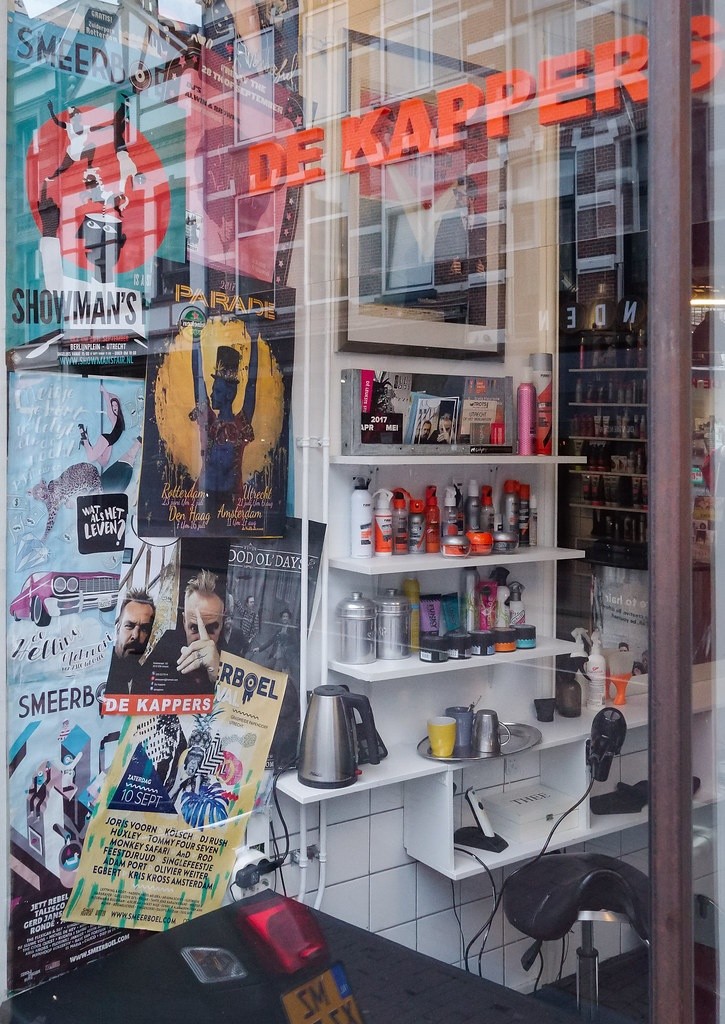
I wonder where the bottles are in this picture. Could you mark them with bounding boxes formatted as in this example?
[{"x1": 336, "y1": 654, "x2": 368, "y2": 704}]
[
  {"x1": 333, "y1": 575, "x2": 421, "y2": 666},
  {"x1": 459, "y1": 567, "x2": 480, "y2": 632},
  {"x1": 517, "y1": 366, "x2": 536, "y2": 456}
]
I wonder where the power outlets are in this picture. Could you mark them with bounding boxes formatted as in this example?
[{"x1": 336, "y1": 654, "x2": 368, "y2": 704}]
[{"x1": 231, "y1": 848, "x2": 274, "y2": 899}]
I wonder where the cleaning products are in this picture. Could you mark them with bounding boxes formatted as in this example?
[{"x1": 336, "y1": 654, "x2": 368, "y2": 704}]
[
  {"x1": 569, "y1": 322, "x2": 649, "y2": 545},
  {"x1": 349, "y1": 464, "x2": 538, "y2": 559},
  {"x1": 514, "y1": 352, "x2": 554, "y2": 456}
]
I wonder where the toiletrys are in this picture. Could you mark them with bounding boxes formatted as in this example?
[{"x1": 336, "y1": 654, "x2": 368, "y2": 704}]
[
  {"x1": 401, "y1": 565, "x2": 537, "y2": 664},
  {"x1": 555, "y1": 626, "x2": 635, "y2": 718}
]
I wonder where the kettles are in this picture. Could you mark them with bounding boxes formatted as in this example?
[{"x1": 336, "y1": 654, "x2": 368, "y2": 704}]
[{"x1": 298, "y1": 685, "x2": 380, "y2": 789}]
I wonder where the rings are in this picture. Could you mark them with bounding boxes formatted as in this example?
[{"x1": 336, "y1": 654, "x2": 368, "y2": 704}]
[
  {"x1": 197, "y1": 649, "x2": 201, "y2": 659},
  {"x1": 198, "y1": 658, "x2": 203, "y2": 667}
]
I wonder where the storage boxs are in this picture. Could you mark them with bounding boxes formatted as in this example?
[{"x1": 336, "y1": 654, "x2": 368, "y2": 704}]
[{"x1": 480, "y1": 783, "x2": 582, "y2": 844}]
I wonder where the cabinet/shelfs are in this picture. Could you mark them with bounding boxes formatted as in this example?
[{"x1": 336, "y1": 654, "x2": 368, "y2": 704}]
[{"x1": 273, "y1": 362, "x2": 715, "y2": 885}]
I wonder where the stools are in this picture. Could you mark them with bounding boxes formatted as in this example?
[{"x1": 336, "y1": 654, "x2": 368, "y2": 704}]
[{"x1": 505, "y1": 845, "x2": 654, "y2": 1019}]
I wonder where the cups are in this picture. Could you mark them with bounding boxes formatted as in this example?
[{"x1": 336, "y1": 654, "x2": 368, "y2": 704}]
[
  {"x1": 471, "y1": 709, "x2": 511, "y2": 753},
  {"x1": 427, "y1": 716, "x2": 456, "y2": 757},
  {"x1": 445, "y1": 706, "x2": 477, "y2": 747}
]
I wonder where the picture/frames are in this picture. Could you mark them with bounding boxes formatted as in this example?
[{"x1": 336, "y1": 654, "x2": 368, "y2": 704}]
[{"x1": 334, "y1": 25, "x2": 510, "y2": 366}]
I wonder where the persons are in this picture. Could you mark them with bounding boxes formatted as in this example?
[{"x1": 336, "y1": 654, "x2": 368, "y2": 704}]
[
  {"x1": 414, "y1": 417, "x2": 454, "y2": 444},
  {"x1": 101, "y1": 569, "x2": 298, "y2": 697}
]
